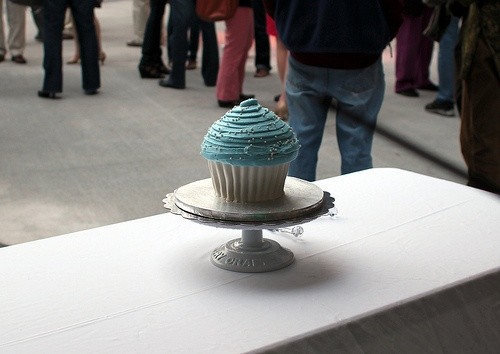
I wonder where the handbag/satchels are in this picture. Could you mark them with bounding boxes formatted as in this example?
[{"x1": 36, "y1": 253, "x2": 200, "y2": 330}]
[
  {"x1": 195, "y1": 0, "x2": 238, "y2": 22},
  {"x1": 421, "y1": 4, "x2": 453, "y2": 42}
]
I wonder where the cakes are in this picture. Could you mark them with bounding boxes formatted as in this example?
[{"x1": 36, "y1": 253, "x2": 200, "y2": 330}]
[{"x1": 200, "y1": 98, "x2": 301, "y2": 202}]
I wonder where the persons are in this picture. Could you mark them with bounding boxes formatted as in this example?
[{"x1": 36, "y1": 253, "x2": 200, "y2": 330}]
[
  {"x1": 267, "y1": 0, "x2": 405, "y2": 182},
  {"x1": 167, "y1": 0, "x2": 200, "y2": 69},
  {"x1": 460, "y1": 0, "x2": 500, "y2": 194},
  {"x1": 11, "y1": 0, "x2": 103, "y2": 98},
  {"x1": 266, "y1": 12, "x2": 290, "y2": 121},
  {"x1": 65, "y1": 7, "x2": 107, "y2": 65},
  {"x1": 424, "y1": 0, "x2": 460, "y2": 116},
  {"x1": 239, "y1": 0, "x2": 272, "y2": 78},
  {"x1": 36, "y1": 7, "x2": 75, "y2": 40},
  {"x1": 138, "y1": 0, "x2": 172, "y2": 79},
  {"x1": 159, "y1": 0, "x2": 220, "y2": 89},
  {"x1": 216, "y1": 0, "x2": 255, "y2": 108},
  {"x1": 395, "y1": 0, "x2": 440, "y2": 98},
  {"x1": 0, "y1": 0, "x2": 29, "y2": 64},
  {"x1": 126, "y1": 0, "x2": 167, "y2": 47}
]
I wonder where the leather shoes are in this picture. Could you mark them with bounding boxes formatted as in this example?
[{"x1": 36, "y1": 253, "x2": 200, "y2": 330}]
[
  {"x1": 36, "y1": 33, "x2": 74, "y2": 41},
  {"x1": 125, "y1": 38, "x2": 145, "y2": 47},
  {"x1": 218, "y1": 93, "x2": 257, "y2": 107},
  {"x1": 0, "y1": 53, "x2": 27, "y2": 63},
  {"x1": 254, "y1": 67, "x2": 268, "y2": 77},
  {"x1": 274, "y1": 92, "x2": 283, "y2": 100}
]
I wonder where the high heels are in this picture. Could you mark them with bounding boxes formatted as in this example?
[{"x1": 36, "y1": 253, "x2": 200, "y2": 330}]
[
  {"x1": 64, "y1": 55, "x2": 85, "y2": 65},
  {"x1": 272, "y1": 103, "x2": 291, "y2": 121},
  {"x1": 96, "y1": 52, "x2": 106, "y2": 65}
]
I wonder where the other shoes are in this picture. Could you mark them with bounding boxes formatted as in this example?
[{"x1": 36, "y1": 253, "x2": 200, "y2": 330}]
[
  {"x1": 396, "y1": 88, "x2": 418, "y2": 97},
  {"x1": 38, "y1": 87, "x2": 56, "y2": 98},
  {"x1": 418, "y1": 84, "x2": 439, "y2": 92},
  {"x1": 157, "y1": 78, "x2": 186, "y2": 88},
  {"x1": 187, "y1": 61, "x2": 197, "y2": 69},
  {"x1": 140, "y1": 60, "x2": 170, "y2": 78}
]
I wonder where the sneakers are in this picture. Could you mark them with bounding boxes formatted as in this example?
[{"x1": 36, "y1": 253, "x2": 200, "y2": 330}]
[{"x1": 425, "y1": 100, "x2": 454, "y2": 116}]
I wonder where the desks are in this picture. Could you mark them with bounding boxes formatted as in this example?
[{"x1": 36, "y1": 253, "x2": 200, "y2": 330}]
[{"x1": 0, "y1": 167, "x2": 500, "y2": 354}]
